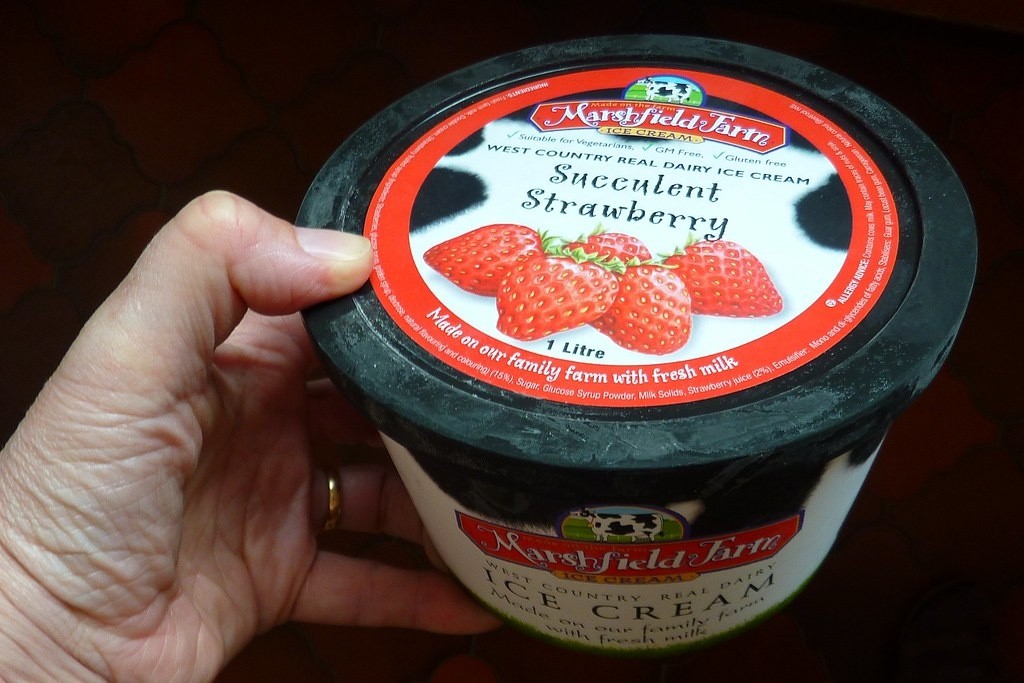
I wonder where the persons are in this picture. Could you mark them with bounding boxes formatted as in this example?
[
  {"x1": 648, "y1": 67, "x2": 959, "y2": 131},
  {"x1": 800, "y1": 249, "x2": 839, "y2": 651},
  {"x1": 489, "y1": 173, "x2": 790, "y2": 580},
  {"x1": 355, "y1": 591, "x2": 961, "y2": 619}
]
[{"x1": 0, "y1": 189, "x2": 503, "y2": 683}]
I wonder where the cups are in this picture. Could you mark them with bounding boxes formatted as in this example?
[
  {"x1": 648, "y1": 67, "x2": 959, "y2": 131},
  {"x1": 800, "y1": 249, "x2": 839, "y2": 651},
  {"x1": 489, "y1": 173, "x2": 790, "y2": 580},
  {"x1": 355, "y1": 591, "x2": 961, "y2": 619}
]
[{"x1": 296, "y1": 33, "x2": 978, "y2": 658}]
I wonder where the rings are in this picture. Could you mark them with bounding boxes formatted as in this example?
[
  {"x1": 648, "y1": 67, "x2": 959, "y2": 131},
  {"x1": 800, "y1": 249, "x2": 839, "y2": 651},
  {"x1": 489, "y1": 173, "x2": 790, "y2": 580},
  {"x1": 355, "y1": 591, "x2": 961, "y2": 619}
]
[{"x1": 326, "y1": 467, "x2": 340, "y2": 531}]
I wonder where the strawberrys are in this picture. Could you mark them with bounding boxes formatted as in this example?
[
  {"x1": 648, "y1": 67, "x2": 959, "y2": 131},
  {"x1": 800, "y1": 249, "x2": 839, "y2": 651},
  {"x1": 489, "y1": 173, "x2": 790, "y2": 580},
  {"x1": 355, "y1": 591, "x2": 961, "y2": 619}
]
[{"x1": 422, "y1": 224, "x2": 784, "y2": 356}]
